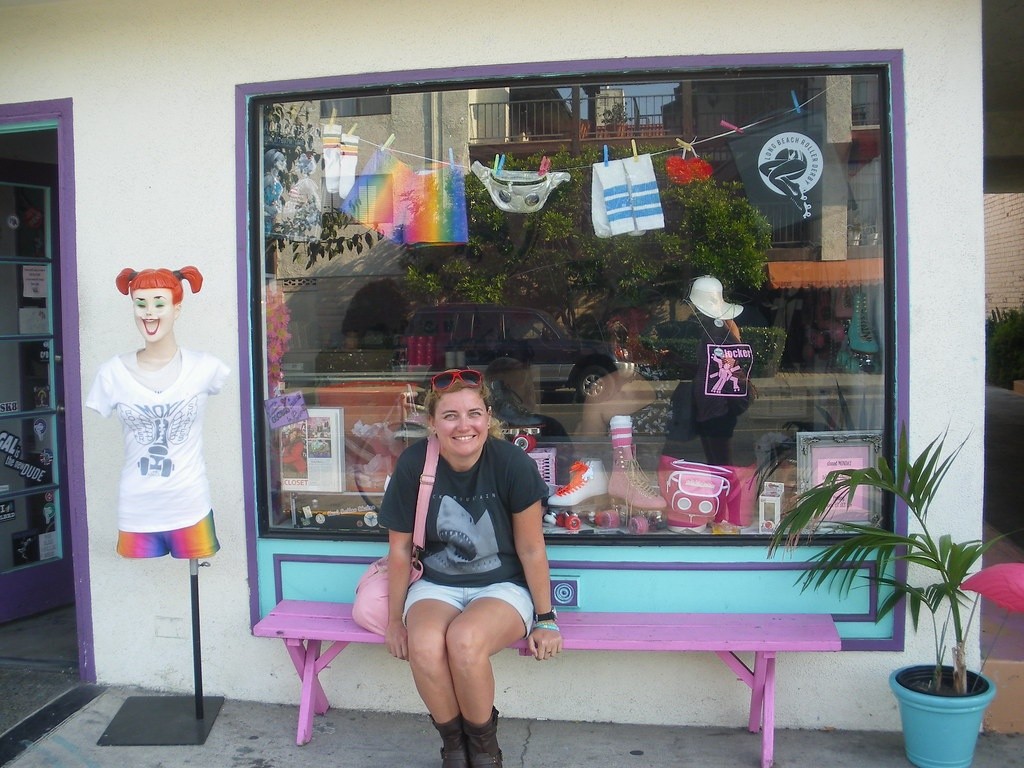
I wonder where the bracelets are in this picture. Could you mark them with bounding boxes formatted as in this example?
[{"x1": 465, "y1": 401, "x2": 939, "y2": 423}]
[{"x1": 533, "y1": 622, "x2": 559, "y2": 631}]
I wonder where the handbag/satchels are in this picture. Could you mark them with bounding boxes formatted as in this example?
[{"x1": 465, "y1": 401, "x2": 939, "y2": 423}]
[
  {"x1": 352, "y1": 555, "x2": 423, "y2": 636},
  {"x1": 657, "y1": 453, "x2": 757, "y2": 527}
]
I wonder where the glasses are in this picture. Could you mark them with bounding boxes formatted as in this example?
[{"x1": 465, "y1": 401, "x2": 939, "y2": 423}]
[{"x1": 431, "y1": 370, "x2": 482, "y2": 392}]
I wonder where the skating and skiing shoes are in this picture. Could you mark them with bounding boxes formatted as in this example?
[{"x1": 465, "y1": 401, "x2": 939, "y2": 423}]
[
  {"x1": 598, "y1": 444, "x2": 667, "y2": 533},
  {"x1": 545, "y1": 459, "x2": 610, "y2": 530}
]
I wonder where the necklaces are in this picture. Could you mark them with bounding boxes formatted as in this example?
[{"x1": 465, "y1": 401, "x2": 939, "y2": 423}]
[{"x1": 683, "y1": 297, "x2": 735, "y2": 356}]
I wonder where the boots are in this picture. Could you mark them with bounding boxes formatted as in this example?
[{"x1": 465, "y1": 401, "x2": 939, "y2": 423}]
[{"x1": 428, "y1": 706, "x2": 504, "y2": 768}]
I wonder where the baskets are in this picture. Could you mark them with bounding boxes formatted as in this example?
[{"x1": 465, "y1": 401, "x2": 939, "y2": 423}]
[{"x1": 526, "y1": 447, "x2": 558, "y2": 485}]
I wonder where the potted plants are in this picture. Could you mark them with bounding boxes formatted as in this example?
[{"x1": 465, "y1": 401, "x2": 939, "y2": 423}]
[{"x1": 762, "y1": 428, "x2": 1024, "y2": 768}]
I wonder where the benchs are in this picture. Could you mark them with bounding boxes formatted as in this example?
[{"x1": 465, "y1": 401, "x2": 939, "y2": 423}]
[{"x1": 252, "y1": 597, "x2": 845, "y2": 768}]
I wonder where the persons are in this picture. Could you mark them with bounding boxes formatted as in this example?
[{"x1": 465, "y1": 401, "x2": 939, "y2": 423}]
[
  {"x1": 484, "y1": 359, "x2": 574, "y2": 485},
  {"x1": 579, "y1": 309, "x2": 692, "y2": 470},
  {"x1": 98, "y1": 266, "x2": 233, "y2": 559},
  {"x1": 263, "y1": 148, "x2": 286, "y2": 234},
  {"x1": 692, "y1": 290, "x2": 744, "y2": 532},
  {"x1": 284, "y1": 153, "x2": 320, "y2": 236},
  {"x1": 342, "y1": 274, "x2": 414, "y2": 347},
  {"x1": 376, "y1": 370, "x2": 563, "y2": 768}
]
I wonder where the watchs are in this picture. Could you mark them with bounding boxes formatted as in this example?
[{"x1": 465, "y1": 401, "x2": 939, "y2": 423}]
[{"x1": 534, "y1": 606, "x2": 557, "y2": 621}]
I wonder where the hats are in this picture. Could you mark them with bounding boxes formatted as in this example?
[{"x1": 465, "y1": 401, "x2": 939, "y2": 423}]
[{"x1": 688, "y1": 276, "x2": 744, "y2": 320}]
